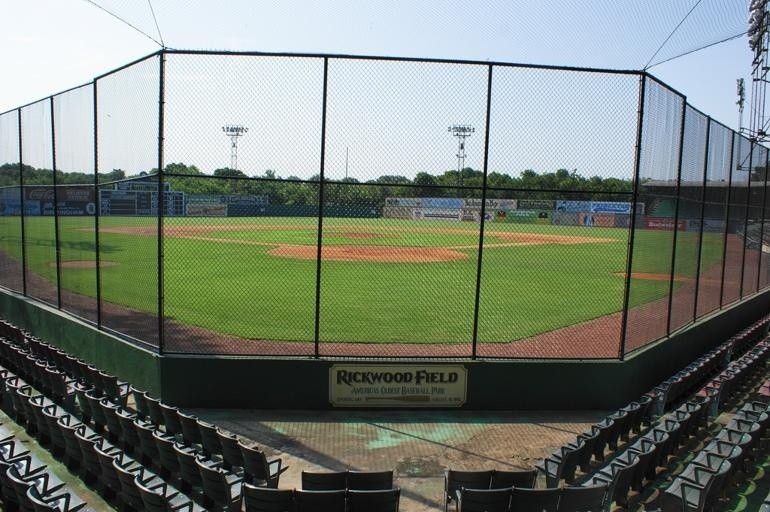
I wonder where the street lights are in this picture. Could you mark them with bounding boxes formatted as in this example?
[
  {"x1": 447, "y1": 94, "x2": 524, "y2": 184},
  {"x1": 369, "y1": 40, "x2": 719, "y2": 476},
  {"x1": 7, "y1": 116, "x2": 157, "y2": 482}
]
[
  {"x1": 222, "y1": 126, "x2": 248, "y2": 172},
  {"x1": 736, "y1": 78, "x2": 745, "y2": 172},
  {"x1": 447, "y1": 124, "x2": 475, "y2": 198}
]
